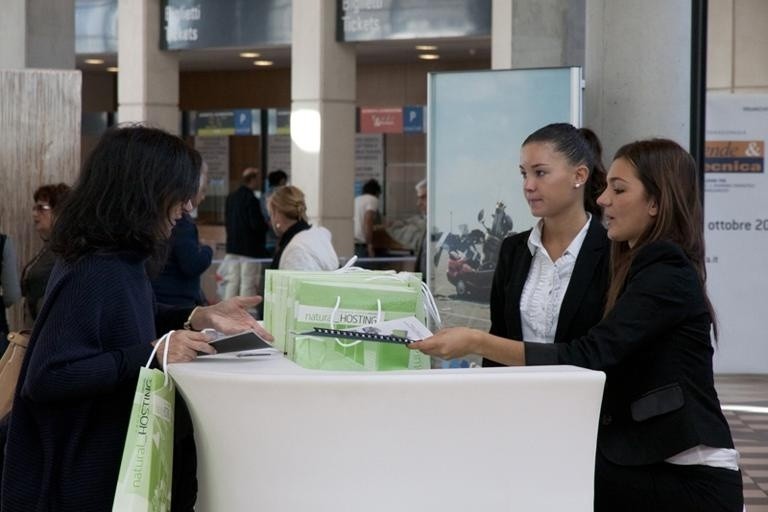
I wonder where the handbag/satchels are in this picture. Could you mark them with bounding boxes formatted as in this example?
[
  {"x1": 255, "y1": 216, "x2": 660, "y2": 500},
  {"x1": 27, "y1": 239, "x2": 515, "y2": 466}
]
[
  {"x1": 111, "y1": 331, "x2": 175, "y2": 511},
  {"x1": 0, "y1": 328, "x2": 34, "y2": 419},
  {"x1": 262, "y1": 255, "x2": 442, "y2": 371}
]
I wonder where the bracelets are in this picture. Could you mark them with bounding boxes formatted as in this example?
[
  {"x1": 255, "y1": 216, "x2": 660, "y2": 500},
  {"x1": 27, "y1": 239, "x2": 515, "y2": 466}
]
[{"x1": 183, "y1": 302, "x2": 202, "y2": 333}]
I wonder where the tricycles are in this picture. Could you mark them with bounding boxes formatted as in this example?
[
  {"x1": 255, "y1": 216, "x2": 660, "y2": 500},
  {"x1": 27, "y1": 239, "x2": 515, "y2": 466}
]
[{"x1": 446, "y1": 201, "x2": 518, "y2": 300}]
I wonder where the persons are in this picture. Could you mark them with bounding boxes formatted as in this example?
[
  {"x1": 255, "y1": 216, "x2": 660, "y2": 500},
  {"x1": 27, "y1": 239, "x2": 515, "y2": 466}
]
[
  {"x1": 223, "y1": 166, "x2": 274, "y2": 319},
  {"x1": 147, "y1": 155, "x2": 218, "y2": 338},
  {"x1": 259, "y1": 169, "x2": 289, "y2": 259},
  {"x1": 354, "y1": 177, "x2": 402, "y2": 257},
  {"x1": 267, "y1": 185, "x2": 341, "y2": 273},
  {"x1": 413, "y1": 177, "x2": 428, "y2": 283},
  {"x1": 406, "y1": 138, "x2": 743, "y2": 512},
  {"x1": 1, "y1": 206, "x2": 23, "y2": 360},
  {"x1": 1, "y1": 125, "x2": 275, "y2": 511},
  {"x1": 18, "y1": 181, "x2": 72, "y2": 325},
  {"x1": 481, "y1": 123, "x2": 614, "y2": 368}
]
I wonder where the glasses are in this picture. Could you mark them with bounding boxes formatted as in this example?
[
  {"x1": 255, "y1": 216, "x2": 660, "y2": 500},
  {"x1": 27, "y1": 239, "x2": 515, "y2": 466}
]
[{"x1": 31, "y1": 204, "x2": 53, "y2": 211}]
